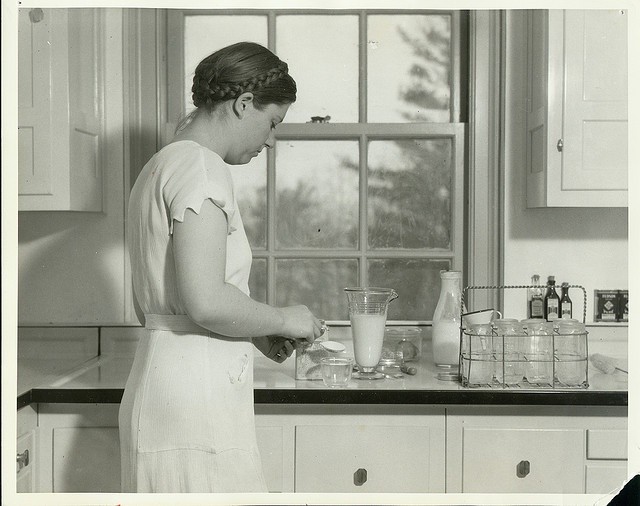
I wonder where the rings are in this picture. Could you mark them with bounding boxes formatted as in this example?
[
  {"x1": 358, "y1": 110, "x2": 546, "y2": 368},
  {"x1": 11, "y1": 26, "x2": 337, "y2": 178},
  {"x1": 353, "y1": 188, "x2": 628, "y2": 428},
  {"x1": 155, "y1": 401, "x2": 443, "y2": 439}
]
[{"x1": 319, "y1": 319, "x2": 329, "y2": 330}]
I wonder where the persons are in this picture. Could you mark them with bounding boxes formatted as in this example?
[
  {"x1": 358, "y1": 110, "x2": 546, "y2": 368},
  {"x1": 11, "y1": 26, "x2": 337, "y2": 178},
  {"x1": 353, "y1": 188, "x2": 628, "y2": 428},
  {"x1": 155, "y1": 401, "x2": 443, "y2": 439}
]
[{"x1": 119, "y1": 41, "x2": 326, "y2": 492}]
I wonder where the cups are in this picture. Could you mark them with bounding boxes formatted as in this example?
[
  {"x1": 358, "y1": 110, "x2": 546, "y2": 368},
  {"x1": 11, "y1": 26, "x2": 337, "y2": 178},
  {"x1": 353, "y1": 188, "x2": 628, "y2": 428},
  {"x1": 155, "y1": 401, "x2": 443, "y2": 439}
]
[
  {"x1": 323, "y1": 358, "x2": 353, "y2": 386},
  {"x1": 462, "y1": 315, "x2": 588, "y2": 387},
  {"x1": 344, "y1": 287, "x2": 397, "y2": 380},
  {"x1": 387, "y1": 327, "x2": 423, "y2": 361},
  {"x1": 294, "y1": 329, "x2": 335, "y2": 380}
]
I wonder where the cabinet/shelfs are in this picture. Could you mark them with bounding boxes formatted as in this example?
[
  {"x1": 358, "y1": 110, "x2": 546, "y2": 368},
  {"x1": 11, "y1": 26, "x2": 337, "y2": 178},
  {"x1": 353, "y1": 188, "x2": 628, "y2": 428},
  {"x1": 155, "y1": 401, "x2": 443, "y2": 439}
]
[
  {"x1": 297, "y1": 392, "x2": 447, "y2": 494},
  {"x1": 527, "y1": 10, "x2": 628, "y2": 208},
  {"x1": 36, "y1": 391, "x2": 297, "y2": 495},
  {"x1": 449, "y1": 391, "x2": 628, "y2": 494},
  {"x1": 17, "y1": 392, "x2": 38, "y2": 494},
  {"x1": 19, "y1": 6, "x2": 109, "y2": 214}
]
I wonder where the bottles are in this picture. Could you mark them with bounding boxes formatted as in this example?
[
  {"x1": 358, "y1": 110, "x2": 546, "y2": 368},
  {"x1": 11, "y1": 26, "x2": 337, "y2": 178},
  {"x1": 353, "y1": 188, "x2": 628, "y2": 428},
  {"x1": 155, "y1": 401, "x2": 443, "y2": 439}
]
[
  {"x1": 430, "y1": 270, "x2": 467, "y2": 372},
  {"x1": 560, "y1": 284, "x2": 575, "y2": 321},
  {"x1": 525, "y1": 271, "x2": 544, "y2": 318},
  {"x1": 545, "y1": 275, "x2": 561, "y2": 322}
]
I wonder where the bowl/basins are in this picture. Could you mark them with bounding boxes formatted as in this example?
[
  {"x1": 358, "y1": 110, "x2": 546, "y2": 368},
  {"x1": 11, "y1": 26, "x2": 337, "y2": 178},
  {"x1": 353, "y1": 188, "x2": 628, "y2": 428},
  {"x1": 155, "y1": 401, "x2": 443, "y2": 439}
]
[{"x1": 322, "y1": 339, "x2": 343, "y2": 357}]
